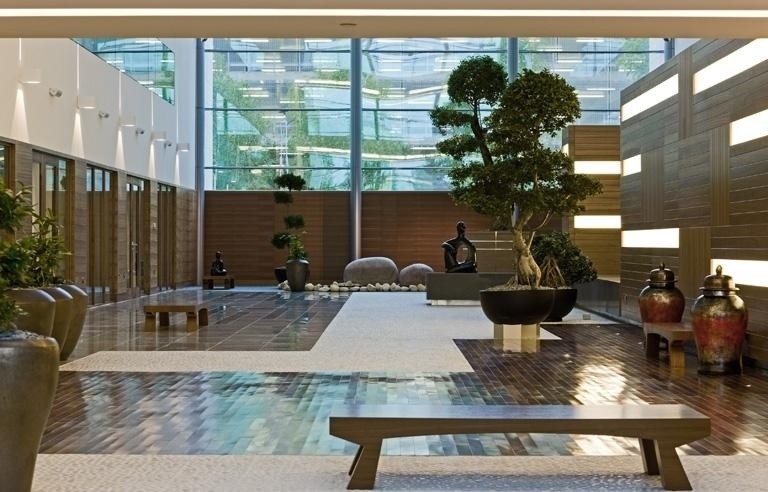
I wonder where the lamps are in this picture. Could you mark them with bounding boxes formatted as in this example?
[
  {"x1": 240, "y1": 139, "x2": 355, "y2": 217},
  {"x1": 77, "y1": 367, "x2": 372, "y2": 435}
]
[{"x1": 149, "y1": 131, "x2": 189, "y2": 152}]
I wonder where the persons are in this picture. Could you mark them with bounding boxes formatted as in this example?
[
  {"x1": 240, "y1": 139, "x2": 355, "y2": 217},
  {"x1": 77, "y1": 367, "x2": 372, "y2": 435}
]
[{"x1": 211, "y1": 249, "x2": 228, "y2": 277}]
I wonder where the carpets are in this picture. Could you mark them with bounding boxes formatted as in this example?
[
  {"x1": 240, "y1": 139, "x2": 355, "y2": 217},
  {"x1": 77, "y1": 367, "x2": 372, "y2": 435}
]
[{"x1": 29, "y1": 455, "x2": 767, "y2": 492}]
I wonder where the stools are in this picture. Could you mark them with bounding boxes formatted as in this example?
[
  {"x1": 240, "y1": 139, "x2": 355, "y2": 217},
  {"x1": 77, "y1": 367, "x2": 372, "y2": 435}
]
[
  {"x1": 201, "y1": 275, "x2": 234, "y2": 289},
  {"x1": 642, "y1": 321, "x2": 693, "y2": 368},
  {"x1": 142, "y1": 301, "x2": 209, "y2": 332}
]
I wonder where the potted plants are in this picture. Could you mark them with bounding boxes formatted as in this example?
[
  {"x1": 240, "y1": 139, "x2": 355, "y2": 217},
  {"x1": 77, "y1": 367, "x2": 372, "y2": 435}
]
[
  {"x1": 268, "y1": 172, "x2": 310, "y2": 292},
  {"x1": 0, "y1": 171, "x2": 88, "y2": 492}
]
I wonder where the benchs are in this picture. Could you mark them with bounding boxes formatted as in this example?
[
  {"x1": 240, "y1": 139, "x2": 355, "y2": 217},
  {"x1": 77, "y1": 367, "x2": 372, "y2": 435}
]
[{"x1": 329, "y1": 405, "x2": 710, "y2": 491}]
